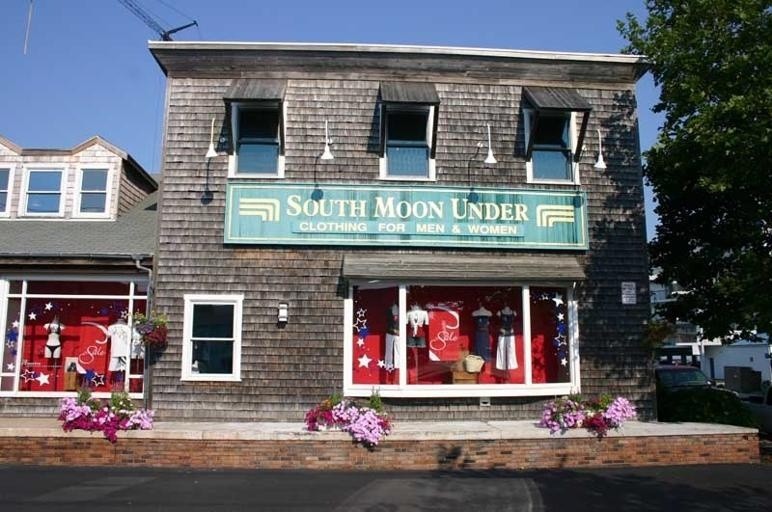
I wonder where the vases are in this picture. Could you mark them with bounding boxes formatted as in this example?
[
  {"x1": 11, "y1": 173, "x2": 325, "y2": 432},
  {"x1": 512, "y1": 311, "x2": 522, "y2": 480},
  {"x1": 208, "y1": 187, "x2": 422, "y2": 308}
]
[
  {"x1": 317, "y1": 421, "x2": 349, "y2": 432},
  {"x1": 572, "y1": 410, "x2": 627, "y2": 427}
]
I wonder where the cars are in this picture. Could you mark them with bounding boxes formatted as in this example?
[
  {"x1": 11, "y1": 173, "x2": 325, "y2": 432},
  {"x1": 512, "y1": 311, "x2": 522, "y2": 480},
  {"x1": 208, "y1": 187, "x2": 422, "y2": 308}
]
[{"x1": 656, "y1": 365, "x2": 739, "y2": 397}]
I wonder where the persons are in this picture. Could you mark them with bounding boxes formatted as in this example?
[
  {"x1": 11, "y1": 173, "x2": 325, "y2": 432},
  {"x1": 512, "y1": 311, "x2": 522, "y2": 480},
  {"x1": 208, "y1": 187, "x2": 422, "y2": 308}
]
[
  {"x1": 472, "y1": 305, "x2": 494, "y2": 363},
  {"x1": 42, "y1": 313, "x2": 65, "y2": 358},
  {"x1": 407, "y1": 302, "x2": 429, "y2": 351},
  {"x1": 494, "y1": 304, "x2": 519, "y2": 369},
  {"x1": 384, "y1": 303, "x2": 402, "y2": 370},
  {"x1": 107, "y1": 318, "x2": 132, "y2": 371}
]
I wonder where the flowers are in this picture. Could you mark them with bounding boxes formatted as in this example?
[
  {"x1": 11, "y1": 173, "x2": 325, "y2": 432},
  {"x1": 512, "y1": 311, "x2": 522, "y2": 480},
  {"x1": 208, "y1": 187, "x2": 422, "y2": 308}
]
[
  {"x1": 306, "y1": 394, "x2": 390, "y2": 446},
  {"x1": 541, "y1": 391, "x2": 637, "y2": 440},
  {"x1": 57, "y1": 386, "x2": 153, "y2": 443}
]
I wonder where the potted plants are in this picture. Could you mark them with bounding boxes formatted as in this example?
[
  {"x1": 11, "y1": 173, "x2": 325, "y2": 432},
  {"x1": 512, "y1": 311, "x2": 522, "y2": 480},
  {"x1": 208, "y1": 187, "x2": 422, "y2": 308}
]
[{"x1": 133, "y1": 310, "x2": 168, "y2": 346}]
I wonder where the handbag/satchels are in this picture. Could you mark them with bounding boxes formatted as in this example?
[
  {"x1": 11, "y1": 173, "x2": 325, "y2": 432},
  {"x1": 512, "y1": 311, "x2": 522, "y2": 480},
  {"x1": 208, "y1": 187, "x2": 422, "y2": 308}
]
[{"x1": 465, "y1": 355, "x2": 483, "y2": 372}]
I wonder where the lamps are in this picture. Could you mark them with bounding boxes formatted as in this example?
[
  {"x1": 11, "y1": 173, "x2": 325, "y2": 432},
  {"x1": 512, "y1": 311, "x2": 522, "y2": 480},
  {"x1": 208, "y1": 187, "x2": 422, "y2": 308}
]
[
  {"x1": 473, "y1": 123, "x2": 498, "y2": 165},
  {"x1": 320, "y1": 119, "x2": 334, "y2": 161},
  {"x1": 592, "y1": 129, "x2": 608, "y2": 170},
  {"x1": 205, "y1": 116, "x2": 220, "y2": 159}
]
